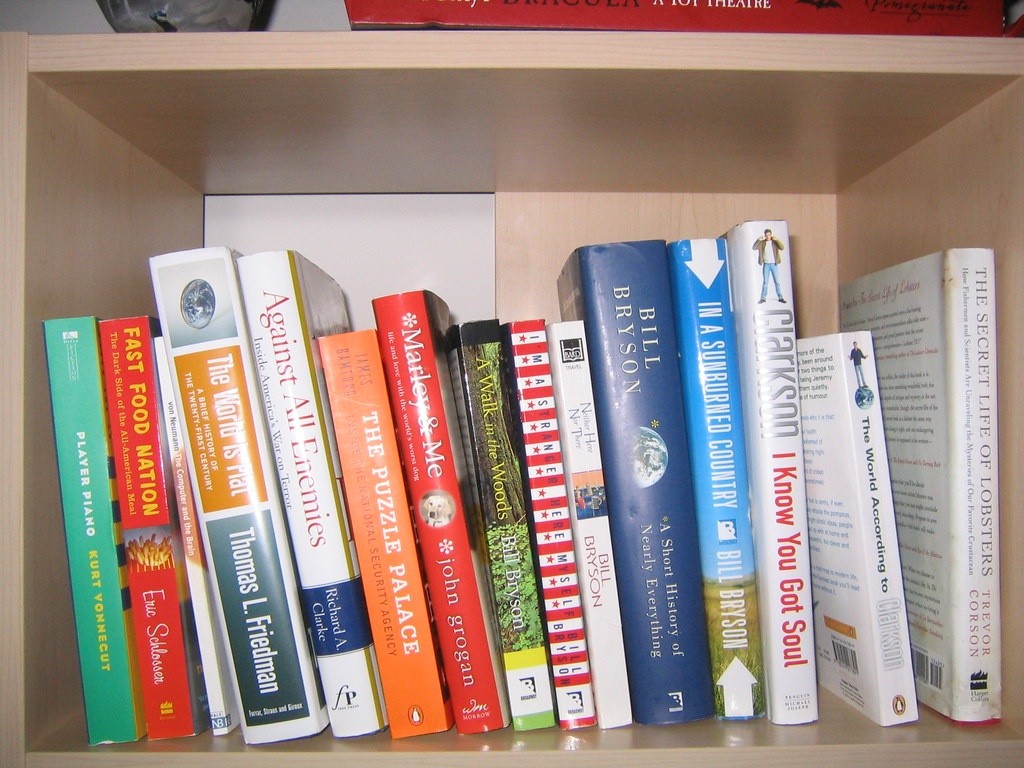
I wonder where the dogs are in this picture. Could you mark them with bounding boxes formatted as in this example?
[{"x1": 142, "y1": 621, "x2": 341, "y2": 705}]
[{"x1": 424, "y1": 495, "x2": 453, "y2": 528}]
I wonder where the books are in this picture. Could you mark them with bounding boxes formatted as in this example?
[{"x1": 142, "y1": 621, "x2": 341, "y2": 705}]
[{"x1": 42, "y1": 222, "x2": 1000, "y2": 746}]
